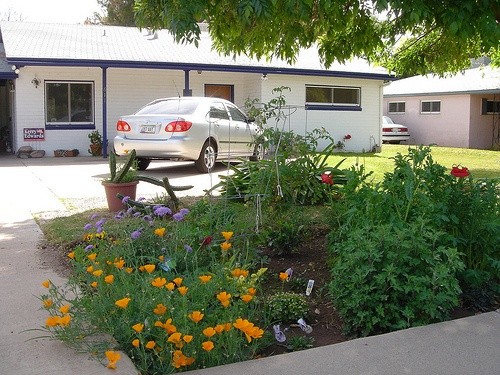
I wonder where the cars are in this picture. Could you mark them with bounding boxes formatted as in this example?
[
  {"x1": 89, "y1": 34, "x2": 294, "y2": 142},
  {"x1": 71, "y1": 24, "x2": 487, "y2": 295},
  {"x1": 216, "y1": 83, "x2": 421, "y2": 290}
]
[
  {"x1": 113, "y1": 95, "x2": 269, "y2": 175},
  {"x1": 382, "y1": 115, "x2": 410, "y2": 144}
]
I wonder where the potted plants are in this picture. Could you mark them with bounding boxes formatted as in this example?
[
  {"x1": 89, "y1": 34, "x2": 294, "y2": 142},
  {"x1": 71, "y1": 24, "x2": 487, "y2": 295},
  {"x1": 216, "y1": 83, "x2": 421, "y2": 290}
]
[
  {"x1": 102, "y1": 149, "x2": 194, "y2": 212},
  {"x1": 88, "y1": 129, "x2": 108, "y2": 157}
]
